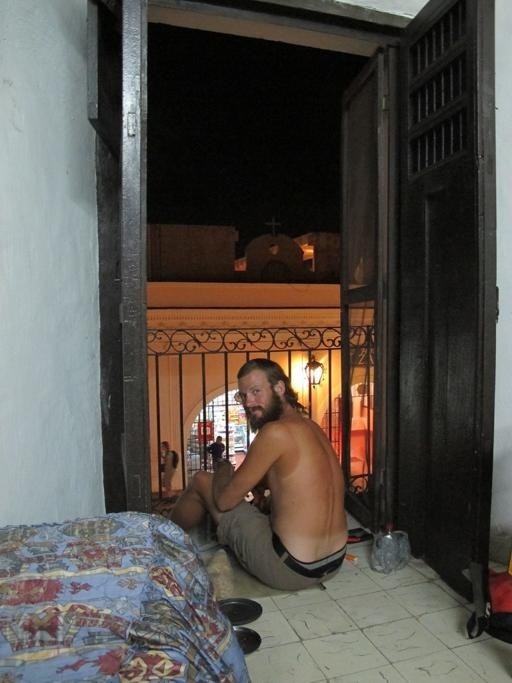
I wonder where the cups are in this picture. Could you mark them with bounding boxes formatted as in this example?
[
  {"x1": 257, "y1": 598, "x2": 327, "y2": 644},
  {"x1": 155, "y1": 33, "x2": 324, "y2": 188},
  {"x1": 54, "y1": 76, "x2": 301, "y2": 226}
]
[{"x1": 371, "y1": 531, "x2": 412, "y2": 574}]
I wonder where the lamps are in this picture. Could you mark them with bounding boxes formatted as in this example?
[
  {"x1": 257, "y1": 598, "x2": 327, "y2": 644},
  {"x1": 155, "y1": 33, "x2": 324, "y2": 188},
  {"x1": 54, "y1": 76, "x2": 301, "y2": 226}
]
[{"x1": 305, "y1": 351, "x2": 324, "y2": 390}]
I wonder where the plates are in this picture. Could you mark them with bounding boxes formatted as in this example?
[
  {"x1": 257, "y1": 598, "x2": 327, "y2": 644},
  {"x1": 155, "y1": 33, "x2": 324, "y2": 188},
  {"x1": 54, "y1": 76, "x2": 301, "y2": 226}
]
[
  {"x1": 218, "y1": 598, "x2": 262, "y2": 626},
  {"x1": 232, "y1": 625, "x2": 262, "y2": 655}
]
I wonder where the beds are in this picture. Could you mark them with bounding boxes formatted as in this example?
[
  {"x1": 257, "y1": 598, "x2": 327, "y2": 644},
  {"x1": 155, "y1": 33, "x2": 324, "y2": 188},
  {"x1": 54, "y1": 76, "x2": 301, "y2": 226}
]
[{"x1": 0, "y1": 511, "x2": 251, "y2": 683}]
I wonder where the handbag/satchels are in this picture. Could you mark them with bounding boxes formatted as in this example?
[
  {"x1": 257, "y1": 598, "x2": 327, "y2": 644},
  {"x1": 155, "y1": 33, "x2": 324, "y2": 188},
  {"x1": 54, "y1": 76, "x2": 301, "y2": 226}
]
[{"x1": 467, "y1": 566, "x2": 511, "y2": 643}]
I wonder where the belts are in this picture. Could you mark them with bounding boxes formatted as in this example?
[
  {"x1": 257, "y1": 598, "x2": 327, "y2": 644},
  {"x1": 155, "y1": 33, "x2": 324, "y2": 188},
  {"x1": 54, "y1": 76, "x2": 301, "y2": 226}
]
[{"x1": 270, "y1": 533, "x2": 346, "y2": 577}]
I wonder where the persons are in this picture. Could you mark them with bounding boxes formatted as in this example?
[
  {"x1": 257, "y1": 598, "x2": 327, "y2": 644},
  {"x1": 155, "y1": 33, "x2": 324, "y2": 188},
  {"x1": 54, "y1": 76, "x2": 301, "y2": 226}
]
[
  {"x1": 207, "y1": 435, "x2": 226, "y2": 470},
  {"x1": 170, "y1": 358, "x2": 349, "y2": 591},
  {"x1": 160, "y1": 441, "x2": 178, "y2": 496}
]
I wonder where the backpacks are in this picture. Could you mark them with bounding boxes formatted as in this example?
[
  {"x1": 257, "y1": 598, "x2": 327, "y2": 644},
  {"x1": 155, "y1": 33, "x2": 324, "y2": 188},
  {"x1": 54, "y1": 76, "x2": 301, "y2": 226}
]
[{"x1": 171, "y1": 450, "x2": 178, "y2": 469}]
